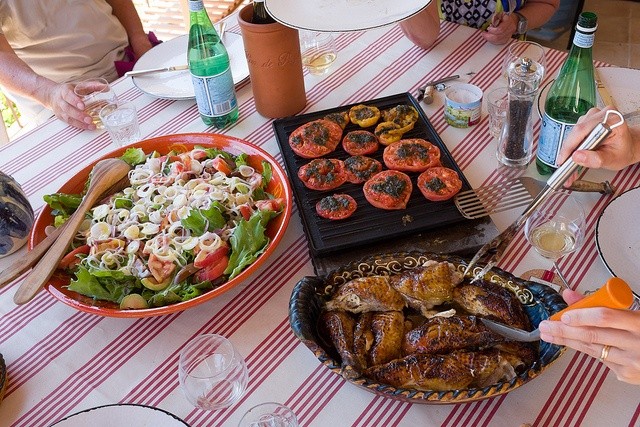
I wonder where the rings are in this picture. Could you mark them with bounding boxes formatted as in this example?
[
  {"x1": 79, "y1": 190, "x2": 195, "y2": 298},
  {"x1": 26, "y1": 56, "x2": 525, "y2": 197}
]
[{"x1": 601, "y1": 344, "x2": 610, "y2": 362}]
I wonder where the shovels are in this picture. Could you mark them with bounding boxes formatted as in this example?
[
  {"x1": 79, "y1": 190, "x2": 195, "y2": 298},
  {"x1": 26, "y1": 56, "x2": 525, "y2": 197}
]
[
  {"x1": 480, "y1": 266, "x2": 634, "y2": 345},
  {"x1": 454, "y1": 177, "x2": 613, "y2": 218}
]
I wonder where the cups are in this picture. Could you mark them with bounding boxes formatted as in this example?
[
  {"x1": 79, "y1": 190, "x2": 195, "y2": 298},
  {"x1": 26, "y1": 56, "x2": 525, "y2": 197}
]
[
  {"x1": 445, "y1": 82, "x2": 483, "y2": 128},
  {"x1": 72, "y1": 76, "x2": 120, "y2": 139},
  {"x1": 502, "y1": 41, "x2": 545, "y2": 84},
  {"x1": 178, "y1": 333, "x2": 250, "y2": 410},
  {"x1": 236, "y1": 2, "x2": 308, "y2": 120},
  {"x1": 298, "y1": 23, "x2": 338, "y2": 79},
  {"x1": 96, "y1": 100, "x2": 151, "y2": 153},
  {"x1": 238, "y1": 399, "x2": 300, "y2": 427},
  {"x1": 525, "y1": 190, "x2": 586, "y2": 260},
  {"x1": 487, "y1": 88, "x2": 508, "y2": 138}
]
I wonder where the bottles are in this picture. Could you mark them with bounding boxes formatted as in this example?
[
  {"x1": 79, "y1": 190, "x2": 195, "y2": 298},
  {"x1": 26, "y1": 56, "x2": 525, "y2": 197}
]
[
  {"x1": 184, "y1": 0, "x2": 242, "y2": 129},
  {"x1": 496, "y1": 58, "x2": 544, "y2": 167},
  {"x1": 534, "y1": 8, "x2": 598, "y2": 183}
]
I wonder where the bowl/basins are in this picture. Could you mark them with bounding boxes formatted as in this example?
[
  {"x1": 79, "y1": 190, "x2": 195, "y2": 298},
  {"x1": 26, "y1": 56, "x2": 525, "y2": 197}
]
[
  {"x1": 286, "y1": 250, "x2": 576, "y2": 406},
  {"x1": 26, "y1": 131, "x2": 294, "y2": 319}
]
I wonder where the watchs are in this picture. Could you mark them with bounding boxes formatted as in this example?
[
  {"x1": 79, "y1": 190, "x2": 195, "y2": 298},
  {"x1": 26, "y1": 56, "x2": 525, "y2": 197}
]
[{"x1": 512, "y1": 10, "x2": 529, "y2": 39}]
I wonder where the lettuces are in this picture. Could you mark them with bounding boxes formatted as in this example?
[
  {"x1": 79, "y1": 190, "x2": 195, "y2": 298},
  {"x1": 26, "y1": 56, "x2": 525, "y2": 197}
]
[{"x1": 43, "y1": 145, "x2": 284, "y2": 307}]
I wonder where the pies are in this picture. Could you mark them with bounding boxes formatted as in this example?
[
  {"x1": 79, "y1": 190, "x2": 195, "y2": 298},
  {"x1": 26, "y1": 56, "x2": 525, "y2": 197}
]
[
  {"x1": 383, "y1": 138, "x2": 440, "y2": 173},
  {"x1": 297, "y1": 158, "x2": 348, "y2": 191},
  {"x1": 288, "y1": 120, "x2": 343, "y2": 158},
  {"x1": 381, "y1": 104, "x2": 419, "y2": 134},
  {"x1": 323, "y1": 111, "x2": 350, "y2": 129},
  {"x1": 374, "y1": 122, "x2": 403, "y2": 145},
  {"x1": 316, "y1": 194, "x2": 358, "y2": 221},
  {"x1": 363, "y1": 170, "x2": 413, "y2": 210},
  {"x1": 349, "y1": 104, "x2": 381, "y2": 128},
  {"x1": 344, "y1": 155, "x2": 383, "y2": 185},
  {"x1": 342, "y1": 130, "x2": 379, "y2": 156},
  {"x1": 417, "y1": 167, "x2": 463, "y2": 202}
]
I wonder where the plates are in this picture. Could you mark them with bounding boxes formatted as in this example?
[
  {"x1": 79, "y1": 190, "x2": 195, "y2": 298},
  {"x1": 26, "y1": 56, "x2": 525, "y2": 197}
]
[
  {"x1": 50, "y1": 403, "x2": 190, "y2": 427},
  {"x1": 258, "y1": 0, "x2": 435, "y2": 35},
  {"x1": 130, "y1": 28, "x2": 266, "y2": 101},
  {"x1": 593, "y1": 185, "x2": 640, "y2": 313},
  {"x1": 537, "y1": 67, "x2": 636, "y2": 122}
]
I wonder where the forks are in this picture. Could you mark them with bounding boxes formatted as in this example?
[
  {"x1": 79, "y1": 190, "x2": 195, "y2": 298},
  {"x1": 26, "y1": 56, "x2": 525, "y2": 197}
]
[
  {"x1": 459, "y1": 102, "x2": 627, "y2": 290},
  {"x1": 477, "y1": 277, "x2": 634, "y2": 343}
]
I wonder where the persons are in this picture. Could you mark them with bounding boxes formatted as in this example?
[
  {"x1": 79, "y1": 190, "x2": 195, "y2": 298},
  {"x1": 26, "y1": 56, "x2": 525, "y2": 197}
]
[
  {"x1": 0, "y1": 0, "x2": 154, "y2": 133},
  {"x1": 401, "y1": 0, "x2": 561, "y2": 51},
  {"x1": 539, "y1": 104, "x2": 640, "y2": 387}
]
